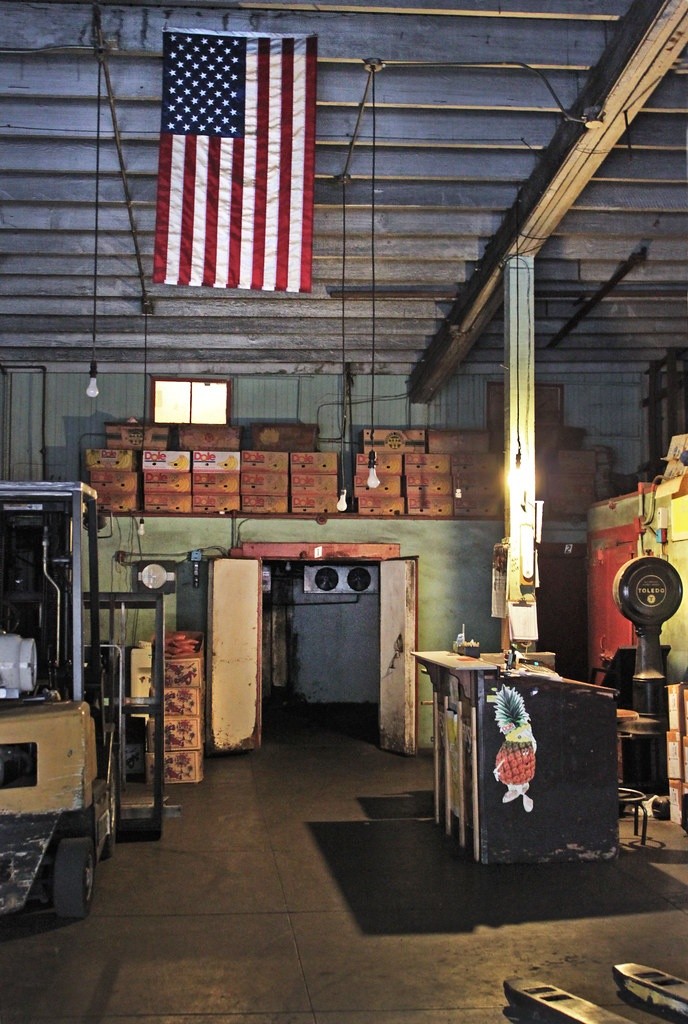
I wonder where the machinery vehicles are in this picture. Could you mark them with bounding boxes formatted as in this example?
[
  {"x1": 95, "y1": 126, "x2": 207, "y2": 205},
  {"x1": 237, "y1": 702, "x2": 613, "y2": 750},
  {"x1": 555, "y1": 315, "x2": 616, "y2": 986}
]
[
  {"x1": 0, "y1": 482, "x2": 115, "y2": 922},
  {"x1": 501, "y1": 960, "x2": 688, "y2": 1024}
]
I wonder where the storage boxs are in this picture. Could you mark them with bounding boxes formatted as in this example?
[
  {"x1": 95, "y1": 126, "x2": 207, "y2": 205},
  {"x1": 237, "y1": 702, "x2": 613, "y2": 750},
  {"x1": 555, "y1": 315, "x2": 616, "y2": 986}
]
[
  {"x1": 661, "y1": 434, "x2": 688, "y2": 461},
  {"x1": 150, "y1": 657, "x2": 202, "y2": 689},
  {"x1": 147, "y1": 717, "x2": 203, "y2": 753},
  {"x1": 105, "y1": 744, "x2": 145, "y2": 774},
  {"x1": 84, "y1": 423, "x2": 612, "y2": 516},
  {"x1": 148, "y1": 686, "x2": 205, "y2": 719},
  {"x1": 145, "y1": 750, "x2": 204, "y2": 786},
  {"x1": 661, "y1": 460, "x2": 688, "y2": 483}
]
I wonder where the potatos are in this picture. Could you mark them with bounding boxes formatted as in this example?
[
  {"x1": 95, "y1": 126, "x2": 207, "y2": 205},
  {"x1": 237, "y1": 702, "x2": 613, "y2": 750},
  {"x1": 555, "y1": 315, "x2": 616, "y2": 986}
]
[{"x1": 165, "y1": 634, "x2": 200, "y2": 656}]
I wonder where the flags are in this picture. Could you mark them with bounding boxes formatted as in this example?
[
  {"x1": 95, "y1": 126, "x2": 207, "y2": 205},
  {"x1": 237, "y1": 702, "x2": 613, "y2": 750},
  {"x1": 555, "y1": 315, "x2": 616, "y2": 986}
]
[{"x1": 153, "y1": 29, "x2": 317, "y2": 293}]
